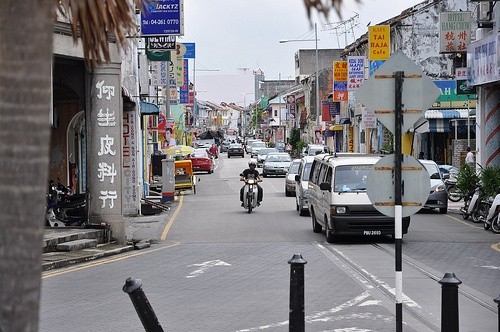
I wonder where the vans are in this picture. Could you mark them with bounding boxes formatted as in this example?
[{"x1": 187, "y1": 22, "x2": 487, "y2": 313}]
[{"x1": 307, "y1": 152, "x2": 411, "y2": 243}]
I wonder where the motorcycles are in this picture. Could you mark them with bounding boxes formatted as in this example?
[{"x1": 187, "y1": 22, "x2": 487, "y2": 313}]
[
  {"x1": 444, "y1": 173, "x2": 500, "y2": 234},
  {"x1": 240, "y1": 174, "x2": 263, "y2": 213},
  {"x1": 46, "y1": 178, "x2": 88, "y2": 227}
]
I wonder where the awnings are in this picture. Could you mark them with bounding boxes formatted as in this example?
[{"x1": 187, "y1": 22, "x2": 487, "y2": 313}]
[
  {"x1": 408, "y1": 108, "x2": 477, "y2": 134},
  {"x1": 139, "y1": 101, "x2": 159, "y2": 113}
]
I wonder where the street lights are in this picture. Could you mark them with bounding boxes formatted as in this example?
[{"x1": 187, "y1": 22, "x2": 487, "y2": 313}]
[
  {"x1": 193, "y1": 68, "x2": 220, "y2": 130},
  {"x1": 279, "y1": 23, "x2": 320, "y2": 125},
  {"x1": 241, "y1": 92, "x2": 258, "y2": 130}
]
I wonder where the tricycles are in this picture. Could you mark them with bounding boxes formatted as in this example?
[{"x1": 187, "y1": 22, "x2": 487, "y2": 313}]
[{"x1": 174, "y1": 160, "x2": 200, "y2": 195}]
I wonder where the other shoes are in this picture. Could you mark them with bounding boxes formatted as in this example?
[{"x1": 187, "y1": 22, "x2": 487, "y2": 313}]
[
  {"x1": 257, "y1": 201, "x2": 260, "y2": 205},
  {"x1": 241, "y1": 203, "x2": 244, "y2": 206}
]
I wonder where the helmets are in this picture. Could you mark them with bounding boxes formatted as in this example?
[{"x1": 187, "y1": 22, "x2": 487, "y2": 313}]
[{"x1": 248, "y1": 159, "x2": 256, "y2": 167}]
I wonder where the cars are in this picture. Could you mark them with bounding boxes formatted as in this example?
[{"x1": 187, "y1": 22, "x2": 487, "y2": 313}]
[
  {"x1": 283, "y1": 144, "x2": 329, "y2": 216},
  {"x1": 256, "y1": 142, "x2": 285, "y2": 168},
  {"x1": 243, "y1": 136, "x2": 266, "y2": 158},
  {"x1": 263, "y1": 152, "x2": 292, "y2": 177},
  {"x1": 184, "y1": 148, "x2": 214, "y2": 174},
  {"x1": 417, "y1": 159, "x2": 453, "y2": 213},
  {"x1": 220, "y1": 139, "x2": 244, "y2": 158},
  {"x1": 197, "y1": 143, "x2": 218, "y2": 160}
]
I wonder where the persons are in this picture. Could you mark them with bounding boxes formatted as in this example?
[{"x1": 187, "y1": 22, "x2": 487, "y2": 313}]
[
  {"x1": 465, "y1": 147, "x2": 474, "y2": 164},
  {"x1": 210, "y1": 144, "x2": 217, "y2": 155},
  {"x1": 286, "y1": 143, "x2": 291, "y2": 154},
  {"x1": 240, "y1": 158, "x2": 263, "y2": 206}
]
